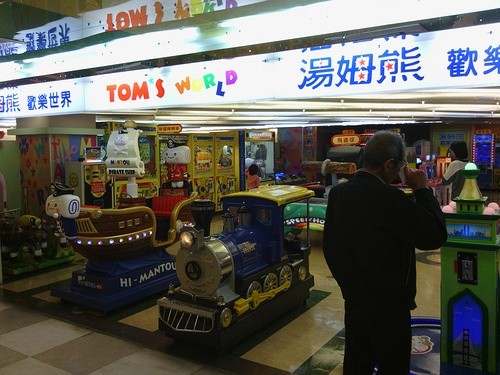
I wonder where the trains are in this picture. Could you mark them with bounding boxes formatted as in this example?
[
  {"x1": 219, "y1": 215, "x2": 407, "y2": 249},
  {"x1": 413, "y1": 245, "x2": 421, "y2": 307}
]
[{"x1": 158, "y1": 184, "x2": 314, "y2": 334}]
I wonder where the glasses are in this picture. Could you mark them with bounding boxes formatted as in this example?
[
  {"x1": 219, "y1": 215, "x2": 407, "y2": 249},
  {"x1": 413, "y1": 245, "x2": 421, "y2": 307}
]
[{"x1": 393, "y1": 159, "x2": 408, "y2": 165}]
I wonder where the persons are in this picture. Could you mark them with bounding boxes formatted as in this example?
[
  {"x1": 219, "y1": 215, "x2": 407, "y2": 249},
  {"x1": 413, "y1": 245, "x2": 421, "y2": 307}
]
[
  {"x1": 245, "y1": 165, "x2": 260, "y2": 190},
  {"x1": 323, "y1": 130, "x2": 448, "y2": 375},
  {"x1": 442, "y1": 141, "x2": 470, "y2": 201}
]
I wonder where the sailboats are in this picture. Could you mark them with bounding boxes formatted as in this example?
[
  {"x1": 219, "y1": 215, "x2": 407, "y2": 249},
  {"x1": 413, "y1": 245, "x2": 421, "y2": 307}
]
[{"x1": 46, "y1": 130, "x2": 198, "y2": 260}]
